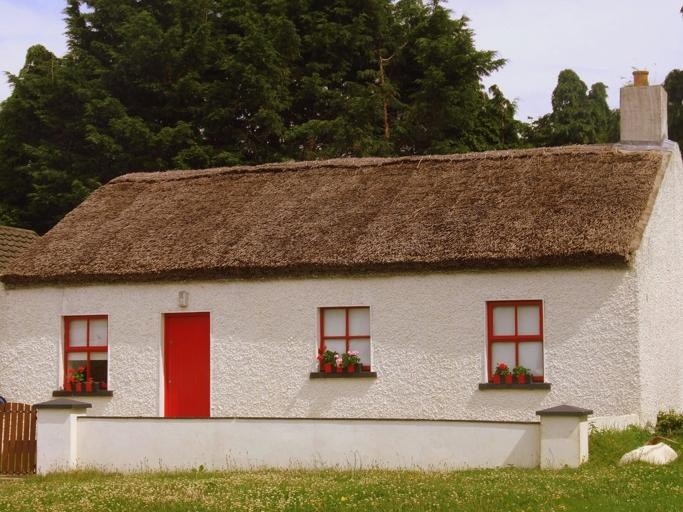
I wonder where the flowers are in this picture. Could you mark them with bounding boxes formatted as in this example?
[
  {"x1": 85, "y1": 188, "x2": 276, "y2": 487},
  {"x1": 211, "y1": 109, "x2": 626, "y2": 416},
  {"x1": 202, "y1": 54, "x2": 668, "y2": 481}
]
[
  {"x1": 65, "y1": 368, "x2": 84, "y2": 382},
  {"x1": 332, "y1": 354, "x2": 345, "y2": 368},
  {"x1": 496, "y1": 363, "x2": 509, "y2": 376},
  {"x1": 69, "y1": 369, "x2": 84, "y2": 383},
  {"x1": 316, "y1": 350, "x2": 335, "y2": 364},
  {"x1": 342, "y1": 351, "x2": 360, "y2": 368}
]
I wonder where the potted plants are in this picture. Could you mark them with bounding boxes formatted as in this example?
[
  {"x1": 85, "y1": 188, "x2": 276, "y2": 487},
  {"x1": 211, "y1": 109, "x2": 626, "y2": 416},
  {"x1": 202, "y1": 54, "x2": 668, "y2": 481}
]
[{"x1": 512, "y1": 366, "x2": 530, "y2": 384}]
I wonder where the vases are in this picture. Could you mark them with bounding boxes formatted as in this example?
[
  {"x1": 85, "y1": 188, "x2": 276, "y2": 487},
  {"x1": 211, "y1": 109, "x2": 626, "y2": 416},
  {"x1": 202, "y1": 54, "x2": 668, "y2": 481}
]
[
  {"x1": 63, "y1": 382, "x2": 72, "y2": 391},
  {"x1": 493, "y1": 375, "x2": 500, "y2": 384},
  {"x1": 85, "y1": 382, "x2": 93, "y2": 393},
  {"x1": 504, "y1": 374, "x2": 512, "y2": 384},
  {"x1": 347, "y1": 365, "x2": 355, "y2": 373},
  {"x1": 74, "y1": 382, "x2": 82, "y2": 392},
  {"x1": 324, "y1": 363, "x2": 332, "y2": 374},
  {"x1": 335, "y1": 367, "x2": 343, "y2": 373}
]
